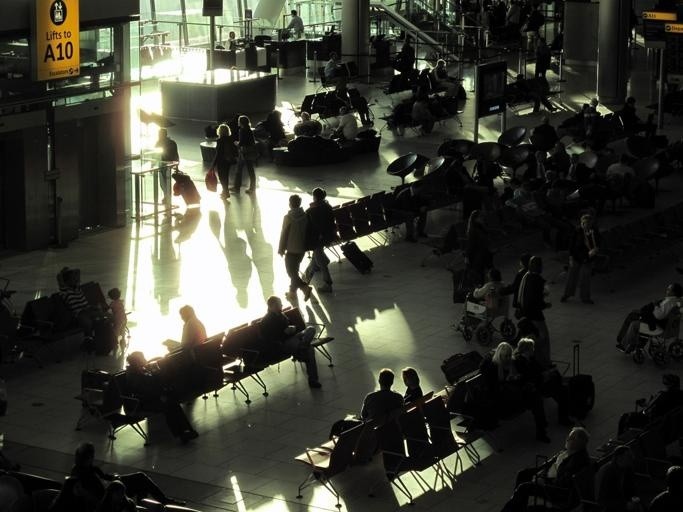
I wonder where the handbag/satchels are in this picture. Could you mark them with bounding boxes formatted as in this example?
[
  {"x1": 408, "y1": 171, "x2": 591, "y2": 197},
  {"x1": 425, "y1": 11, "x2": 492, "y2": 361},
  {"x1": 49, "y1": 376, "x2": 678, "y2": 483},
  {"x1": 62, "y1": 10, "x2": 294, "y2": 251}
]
[{"x1": 205, "y1": 174, "x2": 217, "y2": 192}]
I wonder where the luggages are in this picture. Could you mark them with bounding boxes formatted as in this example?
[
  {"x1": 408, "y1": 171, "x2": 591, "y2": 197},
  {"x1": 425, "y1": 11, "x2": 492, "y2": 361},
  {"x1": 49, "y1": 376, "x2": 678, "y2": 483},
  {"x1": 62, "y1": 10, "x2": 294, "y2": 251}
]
[
  {"x1": 172, "y1": 172, "x2": 201, "y2": 205},
  {"x1": 338, "y1": 240, "x2": 376, "y2": 276},
  {"x1": 562, "y1": 372, "x2": 597, "y2": 421}
]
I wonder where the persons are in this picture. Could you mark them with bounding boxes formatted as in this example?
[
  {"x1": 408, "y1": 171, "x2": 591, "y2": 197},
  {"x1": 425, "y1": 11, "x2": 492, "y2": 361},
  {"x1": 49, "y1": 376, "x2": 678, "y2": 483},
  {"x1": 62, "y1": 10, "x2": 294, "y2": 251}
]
[
  {"x1": 122, "y1": 351, "x2": 199, "y2": 446},
  {"x1": 370, "y1": 1, "x2": 682, "y2": 512},
  {"x1": 284, "y1": 10, "x2": 304, "y2": 34},
  {"x1": 0, "y1": 434, "x2": 186, "y2": 512},
  {"x1": 326, "y1": 367, "x2": 404, "y2": 442},
  {"x1": 275, "y1": 193, "x2": 312, "y2": 302},
  {"x1": 289, "y1": 51, "x2": 373, "y2": 142},
  {"x1": 224, "y1": 31, "x2": 235, "y2": 51},
  {"x1": 76, "y1": 285, "x2": 127, "y2": 358},
  {"x1": 162, "y1": 303, "x2": 207, "y2": 354},
  {"x1": 257, "y1": 294, "x2": 323, "y2": 390},
  {"x1": 149, "y1": 127, "x2": 180, "y2": 205},
  {"x1": 300, "y1": 184, "x2": 336, "y2": 295},
  {"x1": 205, "y1": 108, "x2": 286, "y2": 201}
]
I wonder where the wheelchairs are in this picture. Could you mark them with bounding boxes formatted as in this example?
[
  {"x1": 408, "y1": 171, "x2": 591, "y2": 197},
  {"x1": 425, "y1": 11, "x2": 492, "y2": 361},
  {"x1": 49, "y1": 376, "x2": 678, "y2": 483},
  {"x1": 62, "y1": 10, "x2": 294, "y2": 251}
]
[
  {"x1": 615, "y1": 305, "x2": 683, "y2": 367},
  {"x1": 453, "y1": 283, "x2": 516, "y2": 348}
]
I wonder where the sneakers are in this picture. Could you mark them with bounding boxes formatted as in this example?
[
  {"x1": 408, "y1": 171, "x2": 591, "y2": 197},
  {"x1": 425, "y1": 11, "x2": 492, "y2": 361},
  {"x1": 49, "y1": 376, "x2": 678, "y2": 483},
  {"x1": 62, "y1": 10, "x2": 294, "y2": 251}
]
[
  {"x1": 221, "y1": 192, "x2": 230, "y2": 200},
  {"x1": 302, "y1": 275, "x2": 310, "y2": 285},
  {"x1": 228, "y1": 186, "x2": 241, "y2": 192},
  {"x1": 286, "y1": 292, "x2": 297, "y2": 299},
  {"x1": 317, "y1": 285, "x2": 334, "y2": 294},
  {"x1": 303, "y1": 287, "x2": 312, "y2": 301},
  {"x1": 245, "y1": 189, "x2": 255, "y2": 194}
]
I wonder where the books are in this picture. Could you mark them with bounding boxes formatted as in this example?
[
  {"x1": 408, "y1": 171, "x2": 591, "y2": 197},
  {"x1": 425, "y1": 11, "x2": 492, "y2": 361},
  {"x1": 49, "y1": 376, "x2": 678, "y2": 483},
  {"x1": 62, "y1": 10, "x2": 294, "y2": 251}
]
[{"x1": 161, "y1": 338, "x2": 180, "y2": 351}]
